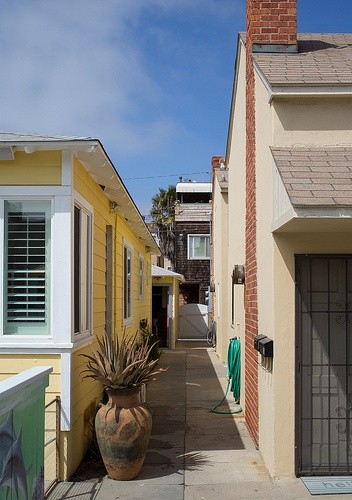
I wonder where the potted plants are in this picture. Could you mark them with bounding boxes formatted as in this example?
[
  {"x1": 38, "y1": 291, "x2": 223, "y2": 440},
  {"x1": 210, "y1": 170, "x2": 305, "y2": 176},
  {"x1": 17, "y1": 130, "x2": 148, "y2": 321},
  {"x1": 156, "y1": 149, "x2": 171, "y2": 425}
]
[{"x1": 77, "y1": 323, "x2": 170, "y2": 481}]
